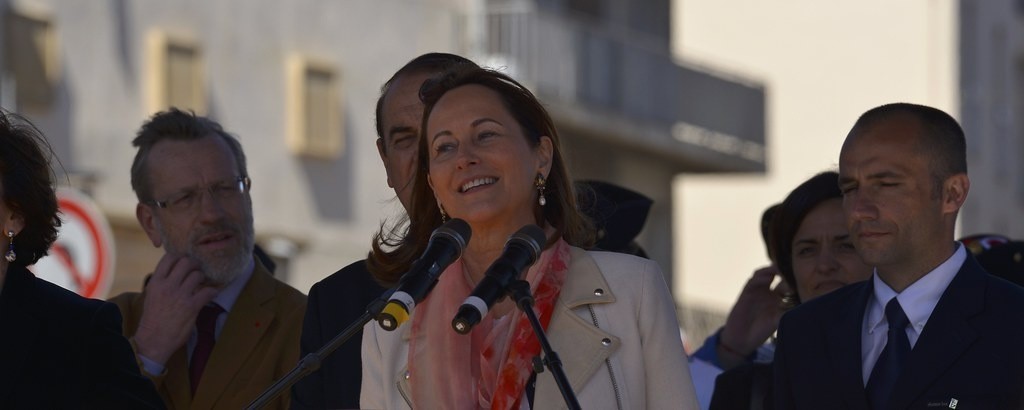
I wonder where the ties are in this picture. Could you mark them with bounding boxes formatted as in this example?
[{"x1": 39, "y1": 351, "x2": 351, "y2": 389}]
[
  {"x1": 188, "y1": 302, "x2": 227, "y2": 400},
  {"x1": 864, "y1": 298, "x2": 912, "y2": 410}
]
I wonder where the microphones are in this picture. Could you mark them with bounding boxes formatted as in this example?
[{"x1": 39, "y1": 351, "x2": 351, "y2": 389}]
[
  {"x1": 452, "y1": 224, "x2": 547, "y2": 335},
  {"x1": 380, "y1": 219, "x2": 471, "y2": 331}
]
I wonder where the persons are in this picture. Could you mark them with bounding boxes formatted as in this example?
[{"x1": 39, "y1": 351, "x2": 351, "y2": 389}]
[
  {"x1": 0, "y1": 111, "x2": 168, "y2": 409},
  {"x1": 769, "y1": 104, "x2": 1023, "y2": 410},
  {"x1": 292, "y1": 52, "x2": 1023, "y2": 410},
  {"x1": 105, "y1": 109, "x2": 307, "y2": 410},
  {"x1": 359, "y1": 70, "x2": 701, "y2": 410}
]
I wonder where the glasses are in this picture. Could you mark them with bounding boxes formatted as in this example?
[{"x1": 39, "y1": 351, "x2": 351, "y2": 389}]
[{"x1": 145, "y1": 174, "x2": 251, "y2": 215}]
